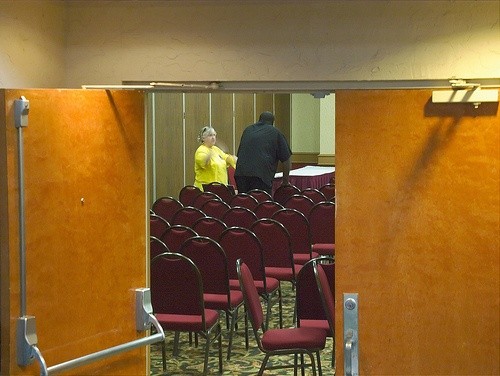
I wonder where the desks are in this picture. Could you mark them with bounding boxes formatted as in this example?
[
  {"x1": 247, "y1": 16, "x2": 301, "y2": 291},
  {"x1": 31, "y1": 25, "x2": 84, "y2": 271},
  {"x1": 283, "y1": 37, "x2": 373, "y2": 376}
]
[{"x1": 271, "y1": 166, "x2": 335, "y2": 192}]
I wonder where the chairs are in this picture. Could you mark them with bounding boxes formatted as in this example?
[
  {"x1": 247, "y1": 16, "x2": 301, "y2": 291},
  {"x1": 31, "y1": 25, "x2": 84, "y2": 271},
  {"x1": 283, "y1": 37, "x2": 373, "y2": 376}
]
[{"x1": 150, "y1": 182, "x2": 335, "y2": 376}]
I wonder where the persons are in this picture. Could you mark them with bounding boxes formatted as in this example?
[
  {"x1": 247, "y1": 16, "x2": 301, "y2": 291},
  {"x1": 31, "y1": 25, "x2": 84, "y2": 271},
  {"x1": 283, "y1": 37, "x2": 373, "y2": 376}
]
[
  {"x1": 234, "y1": 111, "x2": 293, "y2": 196},
  {"x1": 193, "y1": 126, "x2": 237, "y2": 192}
]
